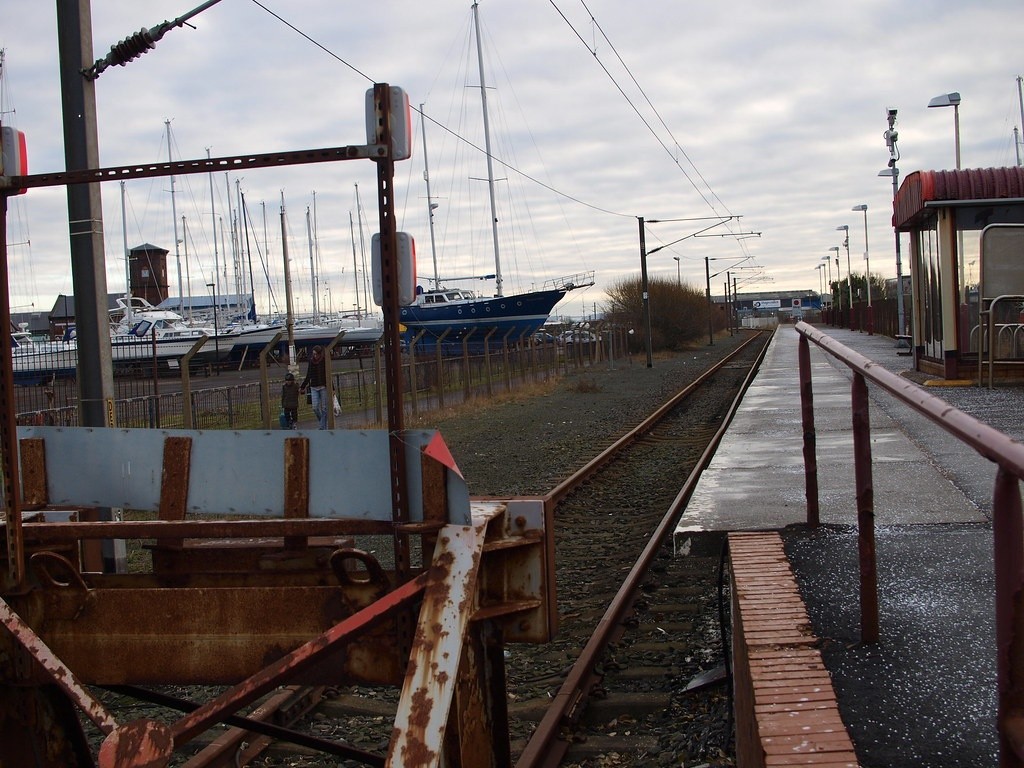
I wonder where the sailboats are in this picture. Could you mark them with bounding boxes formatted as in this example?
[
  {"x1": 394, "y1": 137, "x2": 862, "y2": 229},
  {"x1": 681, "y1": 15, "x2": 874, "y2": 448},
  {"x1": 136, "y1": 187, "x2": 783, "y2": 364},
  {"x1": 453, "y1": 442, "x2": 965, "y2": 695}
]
[{"x1": 1, "y1": 0, "x2": 606, "y2": 388}]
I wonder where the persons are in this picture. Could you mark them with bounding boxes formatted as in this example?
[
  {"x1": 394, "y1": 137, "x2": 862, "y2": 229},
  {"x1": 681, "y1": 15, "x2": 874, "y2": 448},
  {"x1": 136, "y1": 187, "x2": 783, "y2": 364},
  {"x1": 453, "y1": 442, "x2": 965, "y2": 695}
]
[
  {"x1": 280, "y1": 374, "x2": 301, "y2": 429},
  {"x1": 301, "y1": 344, "x2": 337, "y2": 429}
]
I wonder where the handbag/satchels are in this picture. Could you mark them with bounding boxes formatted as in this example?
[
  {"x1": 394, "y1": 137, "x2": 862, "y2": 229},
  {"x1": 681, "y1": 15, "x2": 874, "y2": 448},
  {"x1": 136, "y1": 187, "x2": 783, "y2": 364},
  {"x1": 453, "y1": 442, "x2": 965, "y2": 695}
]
[
  {"x1": 279, "y1": 408, "x2": 287, "y2": 429},
  {"x1": 306, "y1": 381, "x2": 312, "y2": 404},
  {"x1": 333, "y1": 394, "x2": 342, "y2": 417}
]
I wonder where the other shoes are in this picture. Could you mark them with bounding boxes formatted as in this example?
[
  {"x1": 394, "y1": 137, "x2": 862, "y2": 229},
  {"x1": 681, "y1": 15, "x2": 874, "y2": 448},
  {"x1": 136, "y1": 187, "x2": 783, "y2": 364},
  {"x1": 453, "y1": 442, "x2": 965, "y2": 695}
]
[{"x1": 293, "y1": 422, "x2": 297, "y2": 430}]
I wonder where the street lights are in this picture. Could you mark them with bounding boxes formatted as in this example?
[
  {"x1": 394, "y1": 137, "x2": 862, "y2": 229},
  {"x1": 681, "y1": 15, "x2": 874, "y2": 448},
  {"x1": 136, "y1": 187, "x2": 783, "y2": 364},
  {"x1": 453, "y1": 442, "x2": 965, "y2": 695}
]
[
  {"x1": 819, "y1": 262, "x2": 829, "y2": 325},
  {"x1": 834, "y1": 223, "x2": 854, "y2": 332},
  {"x1": 815, "y1": 265, "x2": 826, "y2": 323},
  {"x1": 875, "y1": 107, "x2": 911, "y2": 348},
  {"x1": 927, "y1": 92, "x2": 967, "y2": 306},
  {"x1": 828, "y1": 246, "x2": 845, "y2": 329},
  {"x1": 820, "y1": 256, "x2": 836, "y2": 326},
  {"x1": 851, "y1": 203, "x2": 875, "y2": 336},
  {"x1": 673, "y1": 257, "x2": 681, "y2": 286}
]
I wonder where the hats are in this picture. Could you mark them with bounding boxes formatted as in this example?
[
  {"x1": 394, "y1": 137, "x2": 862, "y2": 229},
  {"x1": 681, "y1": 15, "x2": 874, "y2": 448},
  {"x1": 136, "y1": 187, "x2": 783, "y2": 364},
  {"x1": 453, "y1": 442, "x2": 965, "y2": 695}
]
[{"x1": 284, "y1": 373, "x2": 295, "y2": 382}]
